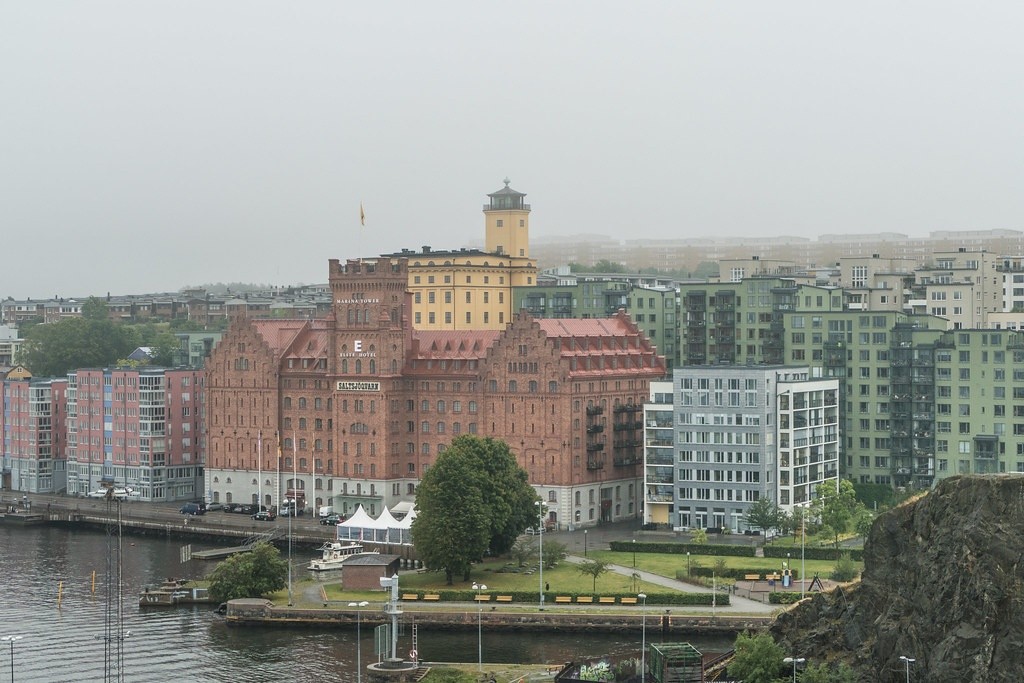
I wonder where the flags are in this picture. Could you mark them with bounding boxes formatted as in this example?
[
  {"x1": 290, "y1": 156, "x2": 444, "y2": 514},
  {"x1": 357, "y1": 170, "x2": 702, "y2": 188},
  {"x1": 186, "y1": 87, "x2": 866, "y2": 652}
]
[{"x1": 360, "y1": 202, "x2": 365, "y2": 227}]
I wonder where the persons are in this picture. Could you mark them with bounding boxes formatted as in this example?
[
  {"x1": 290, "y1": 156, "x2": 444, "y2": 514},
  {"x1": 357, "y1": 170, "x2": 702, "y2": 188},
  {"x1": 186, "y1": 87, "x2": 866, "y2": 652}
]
[{"x1": 544, "y1": 581, "x2": 549, "y2": 592}]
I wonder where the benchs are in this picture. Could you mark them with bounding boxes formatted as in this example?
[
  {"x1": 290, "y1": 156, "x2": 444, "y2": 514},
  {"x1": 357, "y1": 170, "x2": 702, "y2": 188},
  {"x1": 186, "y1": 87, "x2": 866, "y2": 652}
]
[
  {"x1": 496, "y1": 596, "x2": 512, "y2": 603},
  {"x1": 766, "y1": 575, "x2": 780, "y2": 582},
  {"x1": 402, "y1": 594, "x2": 418, "y2": 602},
  {"x1": 577, "y1": 597, "x2": 593, "y2": 605},
  {"x1": 599, "y1": 597, "x2": 615, "y2": 606},
  {"x1": 555, "y1": 597, "x2": 571, "y2": 605},
  {"x1": 424, "y1": 595, "x2": 439, "y2": 602},
  {"x1": 745, "y1": 575, "x2": 762, "y2": 581},
  {"x1": 474, "y1": 595, "x2": 490, "y2": 603},
  {"x1": 621, "y1": 598, "x2": 637, "y2": 606}
]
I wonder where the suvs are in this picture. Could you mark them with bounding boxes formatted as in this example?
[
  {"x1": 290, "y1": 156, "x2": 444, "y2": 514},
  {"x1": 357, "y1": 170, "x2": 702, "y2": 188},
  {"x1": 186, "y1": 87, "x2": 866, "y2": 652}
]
[{"x1": 280, "y1": 505, "x2": 304, "y2": 517}]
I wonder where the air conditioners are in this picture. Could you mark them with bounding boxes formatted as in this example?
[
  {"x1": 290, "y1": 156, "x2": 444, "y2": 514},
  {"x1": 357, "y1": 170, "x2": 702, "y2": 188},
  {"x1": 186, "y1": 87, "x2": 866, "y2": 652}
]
[{"x1": 902, "y1": 342, "x2": 910, "y2": 348}]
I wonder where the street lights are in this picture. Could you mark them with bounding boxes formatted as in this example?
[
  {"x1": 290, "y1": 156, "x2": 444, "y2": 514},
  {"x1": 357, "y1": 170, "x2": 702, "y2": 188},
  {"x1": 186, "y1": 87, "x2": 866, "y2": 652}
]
[
  {"x1": 787, "y1": 553, "x2": 791, "y2": 568},
  {"x1": 535, "y1": 500, "x2": 547, "y2": 609},
  {"x1": 783, "y1": 657, "x2": 806, "y2": 683},
  {"x1": 637, "y1": 593, "x2": 649, "y2": 682},
  {"x1": 686, "y1": 551, "x2": 690, "y2": 580},
  {"x1": 472, "y1": 581, "x2": 487, "y2": 670},
  {"x1": 348, "y1": 601, "x2": 369, "y2": 683},
  {"x1": 632, "y1": 539, "x2": 636, "y2": 567},
  {"x1": 584, "y1": 530, "x2": 588, "y2": 556},
  {"x1": 900, "y1": 656, "x2": 915, "y2": 683}
]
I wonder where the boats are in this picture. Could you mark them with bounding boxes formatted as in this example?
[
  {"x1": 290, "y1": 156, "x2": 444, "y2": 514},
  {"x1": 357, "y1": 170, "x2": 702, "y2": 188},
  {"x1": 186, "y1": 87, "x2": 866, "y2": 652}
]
[{"x1": 306, "y1": 533, "x2": 380, "y2": 571}]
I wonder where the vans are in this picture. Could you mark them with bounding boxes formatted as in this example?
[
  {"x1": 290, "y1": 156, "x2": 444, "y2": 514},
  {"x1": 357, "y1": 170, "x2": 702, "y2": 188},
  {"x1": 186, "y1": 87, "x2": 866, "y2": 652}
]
[
  {"x1": 320, "y1": 506, "x2": 333, "y2": 518},
  {"x1": 180, "y1": 502, "x2": 206, "y2": 516}
]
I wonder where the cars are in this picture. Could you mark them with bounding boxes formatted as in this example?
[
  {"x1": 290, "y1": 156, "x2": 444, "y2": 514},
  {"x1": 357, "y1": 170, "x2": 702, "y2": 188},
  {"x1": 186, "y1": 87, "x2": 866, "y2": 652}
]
[
  {"x1": 319, "y1": 515, "x2": 344, "y2": 527},
  {"x1": 251, "y1": 511, "x2": 276, "y2": 521},
  {"x1": 224, "y1": 502, "x2": 267, "y2": 515},
  {"x1": 204, "y1": 501, "x2": 222, "y2": 513}
]
